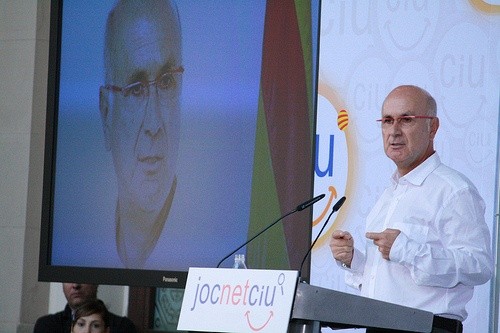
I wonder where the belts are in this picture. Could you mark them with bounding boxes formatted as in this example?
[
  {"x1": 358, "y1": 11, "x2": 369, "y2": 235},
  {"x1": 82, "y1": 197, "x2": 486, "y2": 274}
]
[{"x1": 432, "y1": 314, "x2": 464, "y2": 333}]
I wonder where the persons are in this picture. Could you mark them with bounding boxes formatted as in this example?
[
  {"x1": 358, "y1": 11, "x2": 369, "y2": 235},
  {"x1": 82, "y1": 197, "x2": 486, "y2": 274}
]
[
  {"x1": 33, "y1": 281, "x2": 138, "y2": 333},
  {"x1": 329, "y1": 85, "x2": 492, "y2": 333},
  {"x1": 68, "y1": 0, "x2": 232, "y2": 272}
]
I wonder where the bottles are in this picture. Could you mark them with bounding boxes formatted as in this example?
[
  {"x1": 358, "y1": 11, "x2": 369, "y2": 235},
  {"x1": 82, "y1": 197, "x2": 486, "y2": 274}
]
[{"x1": 233, "y1": 254, "x2": 247, "y2": 269}]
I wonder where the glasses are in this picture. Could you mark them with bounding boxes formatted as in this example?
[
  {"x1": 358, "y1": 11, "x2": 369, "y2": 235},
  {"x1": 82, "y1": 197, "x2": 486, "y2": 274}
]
[
  {"x1": 105, "y1": 69, "x2": 185, "y2": 99},
  {"x1": 377, "y1": 116, "x2": 436, "y2": 128}
]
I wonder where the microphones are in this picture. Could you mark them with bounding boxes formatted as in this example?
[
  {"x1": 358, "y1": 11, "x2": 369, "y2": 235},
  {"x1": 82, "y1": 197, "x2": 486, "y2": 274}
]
[
  {"x1": 216, "y1": 194, "x2": 326, "y2": 268},
  {"x1": 298, "y1": 197, "x2": 347, "y2": 283}
]
